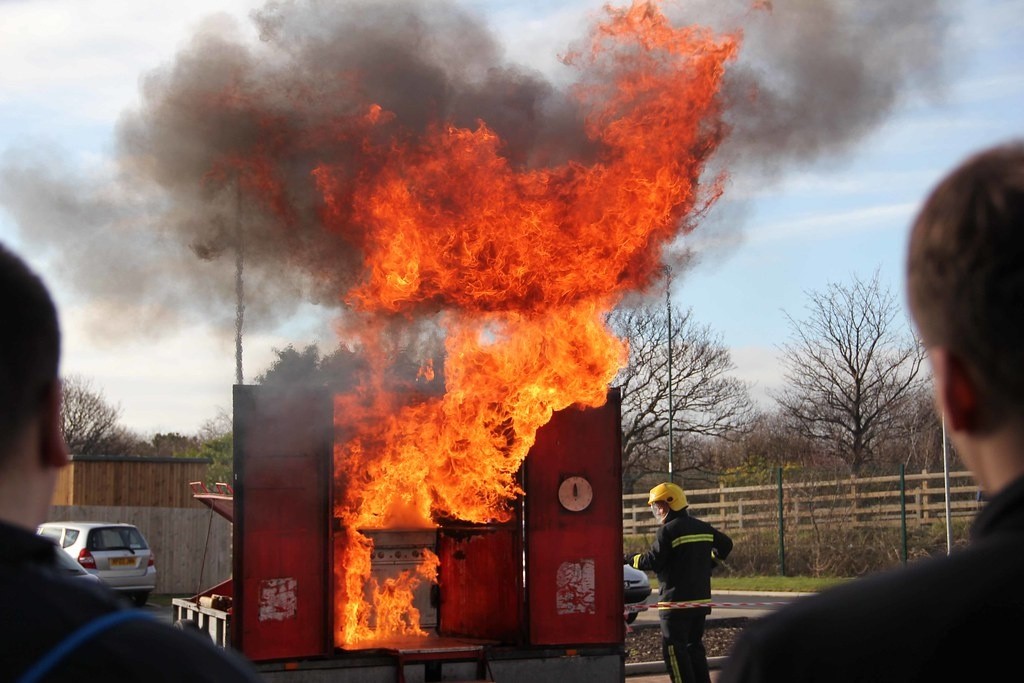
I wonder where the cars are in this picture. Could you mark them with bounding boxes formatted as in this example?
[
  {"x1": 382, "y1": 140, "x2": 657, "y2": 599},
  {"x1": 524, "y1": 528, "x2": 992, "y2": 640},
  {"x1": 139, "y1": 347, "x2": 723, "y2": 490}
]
[{"x1": 623, "y1": 563, "x2": 655, "y2": 625}]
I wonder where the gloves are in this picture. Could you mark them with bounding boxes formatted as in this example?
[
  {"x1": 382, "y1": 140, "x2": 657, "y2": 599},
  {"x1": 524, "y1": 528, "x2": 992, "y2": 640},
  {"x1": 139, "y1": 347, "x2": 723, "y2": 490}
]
[{"x1": 623, "y1": 553, "x2": 639, "y2": 565}]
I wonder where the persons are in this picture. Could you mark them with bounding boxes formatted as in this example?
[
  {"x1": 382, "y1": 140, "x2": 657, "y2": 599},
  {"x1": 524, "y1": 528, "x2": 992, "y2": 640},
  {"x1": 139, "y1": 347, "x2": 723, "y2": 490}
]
[
  {"x1": 714, "y1": 131, "x2": 1024, "y2": 683},
  {"x1": 621, "y1": 480, "x2": 734, "y2": 683},
  {"x1": 1, "y1": 239, "x2": 265, "y2": 683}
]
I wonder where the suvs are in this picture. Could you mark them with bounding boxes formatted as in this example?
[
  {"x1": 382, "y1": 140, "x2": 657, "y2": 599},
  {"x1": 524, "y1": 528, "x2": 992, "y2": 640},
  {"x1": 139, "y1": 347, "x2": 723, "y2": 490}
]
[{"x1": 38, "y1": 521, "x2": 159, "y2": 607}]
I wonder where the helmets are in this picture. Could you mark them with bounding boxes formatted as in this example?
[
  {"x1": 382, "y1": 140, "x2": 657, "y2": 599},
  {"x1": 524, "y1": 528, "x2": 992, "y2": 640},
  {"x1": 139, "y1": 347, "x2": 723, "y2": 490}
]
[{"x1": 647, "y1": 482, "x2": 689, "y2": 511}]
